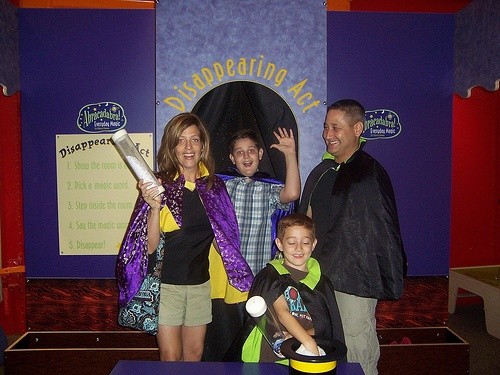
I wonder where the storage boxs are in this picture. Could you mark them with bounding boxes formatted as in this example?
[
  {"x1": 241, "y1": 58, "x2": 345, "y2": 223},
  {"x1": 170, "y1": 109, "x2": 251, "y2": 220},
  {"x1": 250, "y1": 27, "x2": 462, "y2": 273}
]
[
  {"x1": 376, "y1": 326, "x2": 470, "y2": 375},
  {"x1": 3, "y1": 330, "x2": 160, "y2": 375}
]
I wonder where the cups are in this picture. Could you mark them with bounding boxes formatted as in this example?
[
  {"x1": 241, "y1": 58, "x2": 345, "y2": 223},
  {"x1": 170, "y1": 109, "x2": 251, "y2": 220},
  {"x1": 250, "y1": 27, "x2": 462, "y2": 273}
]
[
  {"x1": 112, "y1": 129, "x2": 165, "y2": 199},
  {"x1": 246, "y1": 296, "x2": 286, "y2": 358}
]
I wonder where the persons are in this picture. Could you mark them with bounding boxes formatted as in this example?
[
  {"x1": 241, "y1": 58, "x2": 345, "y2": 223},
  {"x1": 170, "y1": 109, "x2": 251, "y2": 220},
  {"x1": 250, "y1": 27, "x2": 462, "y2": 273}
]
[
  {"x1": 240, "y1": 214, "x2": 348, "y2": 364},
  {"x1": 115, "y1": 112, "x2": 255, "y2": 362},
  {"x1": 213, "y1": 127, "x2": 302, "y2": 361},
  {"x1": 295, "y1": 99, "x2": 408, "y2": 375}
]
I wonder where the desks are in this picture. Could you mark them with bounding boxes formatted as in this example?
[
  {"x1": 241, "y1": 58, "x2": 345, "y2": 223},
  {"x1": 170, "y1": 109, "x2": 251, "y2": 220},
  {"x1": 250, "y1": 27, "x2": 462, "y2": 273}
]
[{"x1": 109, "y1": 360, "x2": 365, "y2": 375}]
